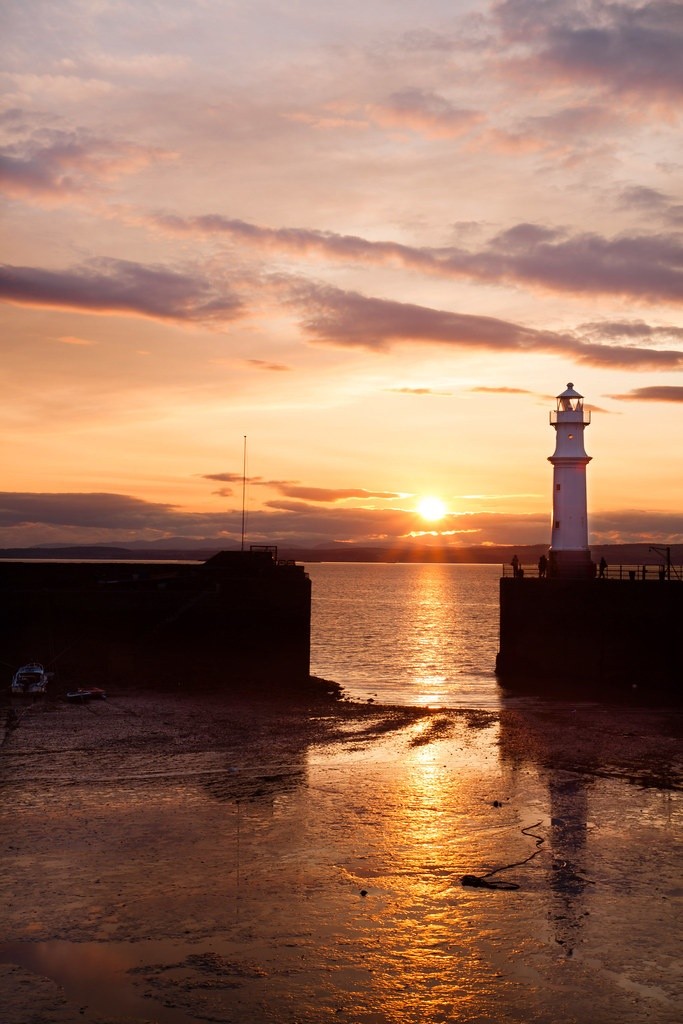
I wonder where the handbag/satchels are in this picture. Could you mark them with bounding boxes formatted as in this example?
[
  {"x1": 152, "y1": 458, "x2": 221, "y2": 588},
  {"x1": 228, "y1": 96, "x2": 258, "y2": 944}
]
[{"x1": 511, "y1": 561, "x2": 514, "y2": 567}]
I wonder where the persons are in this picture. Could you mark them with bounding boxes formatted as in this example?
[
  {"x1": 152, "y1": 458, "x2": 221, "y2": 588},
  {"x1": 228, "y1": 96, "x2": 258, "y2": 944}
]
[
  {"x1": 538, "y1": 555, "x2": 546, "y2": 577},
  {"x1": 598, "y1": 556, "x2": 608, "y2": 578},
  {"x1": 510, "y1": 555, "x2": 520, "y2": 577}
]
